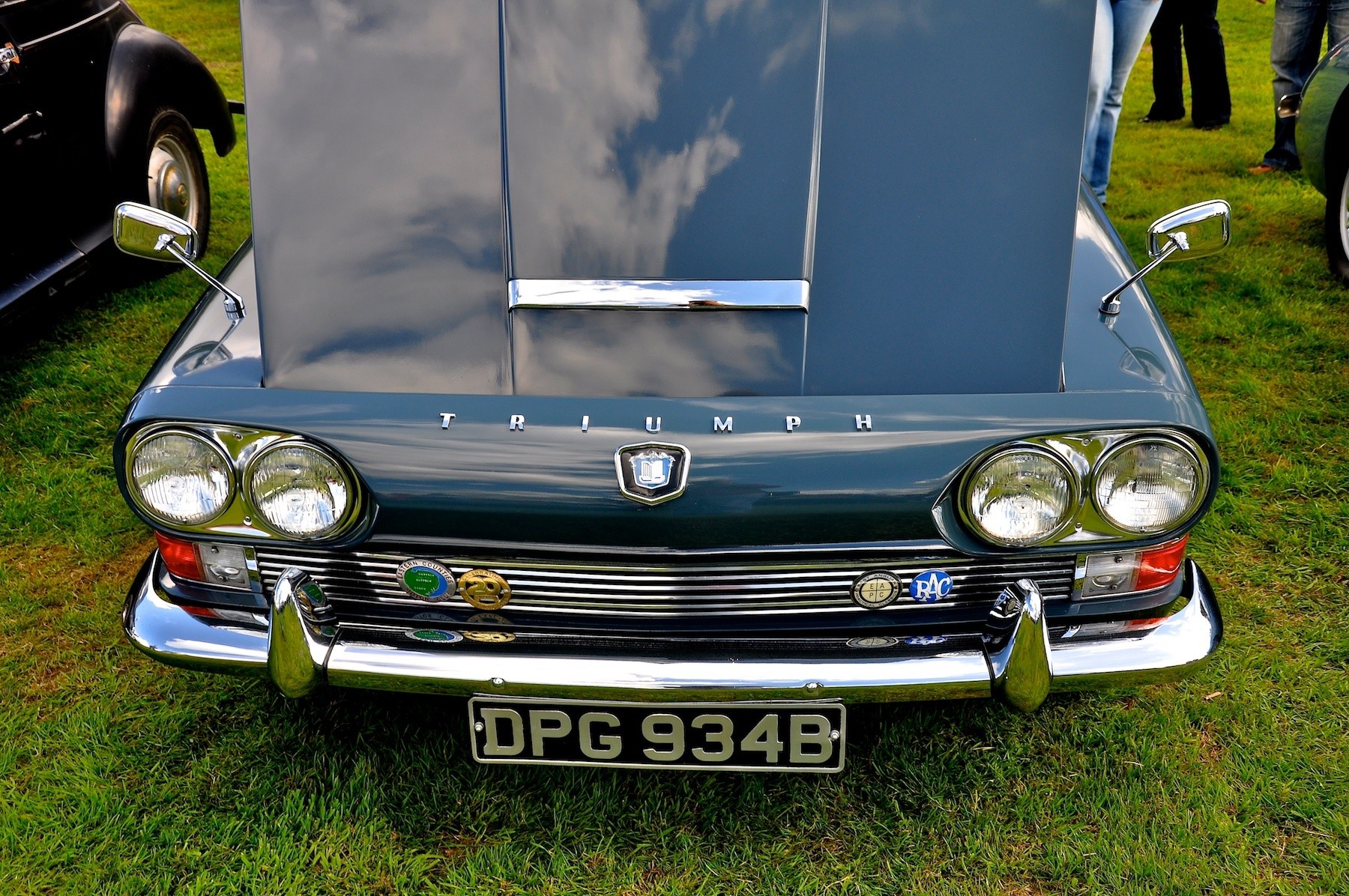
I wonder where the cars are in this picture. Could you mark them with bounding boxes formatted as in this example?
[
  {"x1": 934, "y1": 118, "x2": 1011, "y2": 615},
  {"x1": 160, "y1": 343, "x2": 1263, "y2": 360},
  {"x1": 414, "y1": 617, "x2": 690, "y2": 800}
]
[
  {"x1": 100, "y1": 0, "x2": 1235, "y2": 778},
  {"x1": 0, "y1": 0, "x2": 246, "y2": 372}
]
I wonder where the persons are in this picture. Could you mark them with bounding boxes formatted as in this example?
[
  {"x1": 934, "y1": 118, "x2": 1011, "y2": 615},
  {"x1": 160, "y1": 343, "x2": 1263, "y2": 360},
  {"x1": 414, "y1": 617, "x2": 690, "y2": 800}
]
[
  {"x1": 1079, "y1": 0, "x2": 1164, "y2": 208},
  {"x1": 1240, "y1": 0, "x2": 1349, "y2": 176},
  {"x1": 1138, "y1": 0, "x2": 1231, "y2": 132}
]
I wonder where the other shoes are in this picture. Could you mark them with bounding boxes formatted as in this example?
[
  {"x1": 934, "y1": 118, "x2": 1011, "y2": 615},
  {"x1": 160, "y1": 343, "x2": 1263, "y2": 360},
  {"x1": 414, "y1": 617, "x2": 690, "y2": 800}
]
[
  {"x1": 1138, "y1": 116, "x2": 1158, "y2": 122},
  {"x1": 1246, "y1": 164, "x2": 1275, "y2": 175}
]
